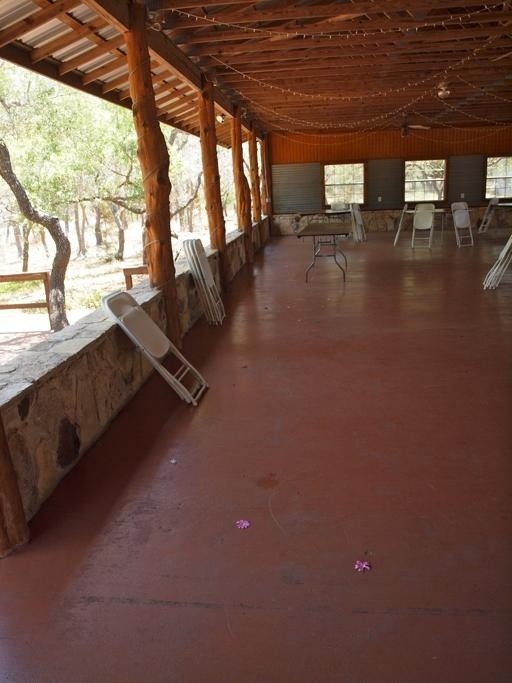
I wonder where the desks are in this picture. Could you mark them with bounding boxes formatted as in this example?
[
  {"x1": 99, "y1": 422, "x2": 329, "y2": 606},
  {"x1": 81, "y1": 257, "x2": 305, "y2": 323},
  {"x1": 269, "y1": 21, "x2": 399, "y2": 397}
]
[
  {"x1": 298, "y1": 208, "x2": 351, "y2": 252},
  {"x1": 296, "y1": 222, "x2": 352, "y2": 283}
]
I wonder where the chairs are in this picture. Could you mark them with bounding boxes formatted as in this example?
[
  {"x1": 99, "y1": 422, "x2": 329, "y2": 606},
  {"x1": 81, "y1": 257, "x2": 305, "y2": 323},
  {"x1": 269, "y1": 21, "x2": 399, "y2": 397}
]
[
  {"x1": 102, "y1": 237, "x2": 228, "y2": 407},
  {"x1": 330, "y1": 201, "x2": 369, "y2": 244},
  {"x1": 390, "y1": 196, "x2": 512, "y2": 291}
]
